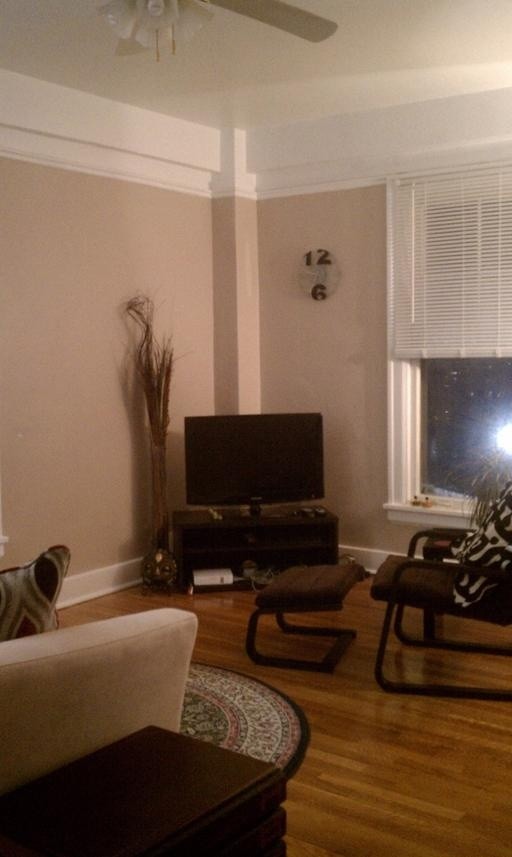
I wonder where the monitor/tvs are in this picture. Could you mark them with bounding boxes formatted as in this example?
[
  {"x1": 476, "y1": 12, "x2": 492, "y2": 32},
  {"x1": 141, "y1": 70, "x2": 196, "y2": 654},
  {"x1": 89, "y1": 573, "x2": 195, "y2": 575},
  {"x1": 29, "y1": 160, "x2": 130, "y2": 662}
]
[{"x1": 184, "y1": 413, "x2": 325, "y2": 519}]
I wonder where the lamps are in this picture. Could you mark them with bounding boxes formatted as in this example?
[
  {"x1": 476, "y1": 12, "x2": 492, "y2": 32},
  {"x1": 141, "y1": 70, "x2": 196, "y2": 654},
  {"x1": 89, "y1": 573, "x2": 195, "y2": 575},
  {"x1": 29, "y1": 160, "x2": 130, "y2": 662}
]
[{"x1": 97, "y1": 0, "x2": 214, "y2": 59}]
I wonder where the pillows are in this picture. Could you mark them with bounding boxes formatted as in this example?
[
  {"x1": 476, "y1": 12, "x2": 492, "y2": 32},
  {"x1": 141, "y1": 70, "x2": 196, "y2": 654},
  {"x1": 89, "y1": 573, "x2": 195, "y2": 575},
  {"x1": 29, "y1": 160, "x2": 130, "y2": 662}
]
[
  {"x1": 451, "y1": 480, "x2": 512, "y2": 613},
  {"x1": 0, "y1": 545, "x2": 71, "y2": 642}
]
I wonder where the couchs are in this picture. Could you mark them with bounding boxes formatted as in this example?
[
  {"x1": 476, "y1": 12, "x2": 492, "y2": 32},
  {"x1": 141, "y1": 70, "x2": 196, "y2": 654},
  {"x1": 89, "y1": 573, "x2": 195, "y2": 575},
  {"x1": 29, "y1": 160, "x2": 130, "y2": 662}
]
[{"x1": 0, "y1": 607, "x2": 199, "y2": 796}]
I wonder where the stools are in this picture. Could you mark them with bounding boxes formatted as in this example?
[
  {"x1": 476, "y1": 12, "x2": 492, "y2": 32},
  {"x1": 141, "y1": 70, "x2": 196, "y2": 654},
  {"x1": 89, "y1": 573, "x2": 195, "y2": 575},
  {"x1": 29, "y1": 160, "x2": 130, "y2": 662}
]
[{"x1": 245, "y1": 565, "x2": 370, "y2": 674}]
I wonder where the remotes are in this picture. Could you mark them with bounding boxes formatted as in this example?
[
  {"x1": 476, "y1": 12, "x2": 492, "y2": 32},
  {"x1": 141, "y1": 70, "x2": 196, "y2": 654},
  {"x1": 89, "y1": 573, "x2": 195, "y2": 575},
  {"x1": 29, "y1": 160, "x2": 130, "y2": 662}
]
[{"x1": 312, "y1": 504, "x2": 325, "y2": 515}]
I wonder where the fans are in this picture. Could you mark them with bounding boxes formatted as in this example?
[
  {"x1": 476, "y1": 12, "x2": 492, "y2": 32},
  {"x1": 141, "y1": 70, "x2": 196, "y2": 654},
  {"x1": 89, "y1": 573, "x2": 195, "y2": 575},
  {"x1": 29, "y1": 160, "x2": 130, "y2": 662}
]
[{"x1": 204, "y1": 0, "x2": 337, "y2": 43}]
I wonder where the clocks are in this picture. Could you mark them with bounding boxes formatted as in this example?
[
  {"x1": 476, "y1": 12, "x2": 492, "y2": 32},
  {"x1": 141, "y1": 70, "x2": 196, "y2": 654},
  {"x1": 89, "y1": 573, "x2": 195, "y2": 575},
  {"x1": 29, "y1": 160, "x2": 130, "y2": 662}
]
[{"x1": 296, "y1": 249, "x2": 339, "y2": 301}]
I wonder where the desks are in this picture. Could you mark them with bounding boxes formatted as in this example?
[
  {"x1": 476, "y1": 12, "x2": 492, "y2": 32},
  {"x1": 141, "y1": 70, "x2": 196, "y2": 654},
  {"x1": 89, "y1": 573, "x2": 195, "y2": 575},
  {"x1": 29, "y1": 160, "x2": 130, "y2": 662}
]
[{"x1": 0, "y1": 725, "x2": 288, "y2": 857}]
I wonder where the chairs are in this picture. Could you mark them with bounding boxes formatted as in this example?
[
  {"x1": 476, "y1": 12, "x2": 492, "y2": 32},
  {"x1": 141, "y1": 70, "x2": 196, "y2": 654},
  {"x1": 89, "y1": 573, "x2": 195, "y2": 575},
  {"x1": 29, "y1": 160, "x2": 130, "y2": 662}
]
[{"x1": 370, "y1": 476, "x2": 512, "y2": 703}]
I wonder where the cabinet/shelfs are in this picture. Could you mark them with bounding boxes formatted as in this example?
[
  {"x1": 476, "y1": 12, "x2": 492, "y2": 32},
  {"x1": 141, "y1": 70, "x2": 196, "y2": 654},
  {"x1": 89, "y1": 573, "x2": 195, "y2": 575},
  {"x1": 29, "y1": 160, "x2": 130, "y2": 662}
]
[{"x1": 171, "y1": 503, "x2": 339, "y2": 595}]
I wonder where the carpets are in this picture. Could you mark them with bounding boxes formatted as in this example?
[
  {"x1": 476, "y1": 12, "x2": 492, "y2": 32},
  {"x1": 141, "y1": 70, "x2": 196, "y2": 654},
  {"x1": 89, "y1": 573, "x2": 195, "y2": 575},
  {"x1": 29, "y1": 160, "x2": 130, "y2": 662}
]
[{"x1": 179, "y1": 660, "x2": 310, "y2": 780}]
